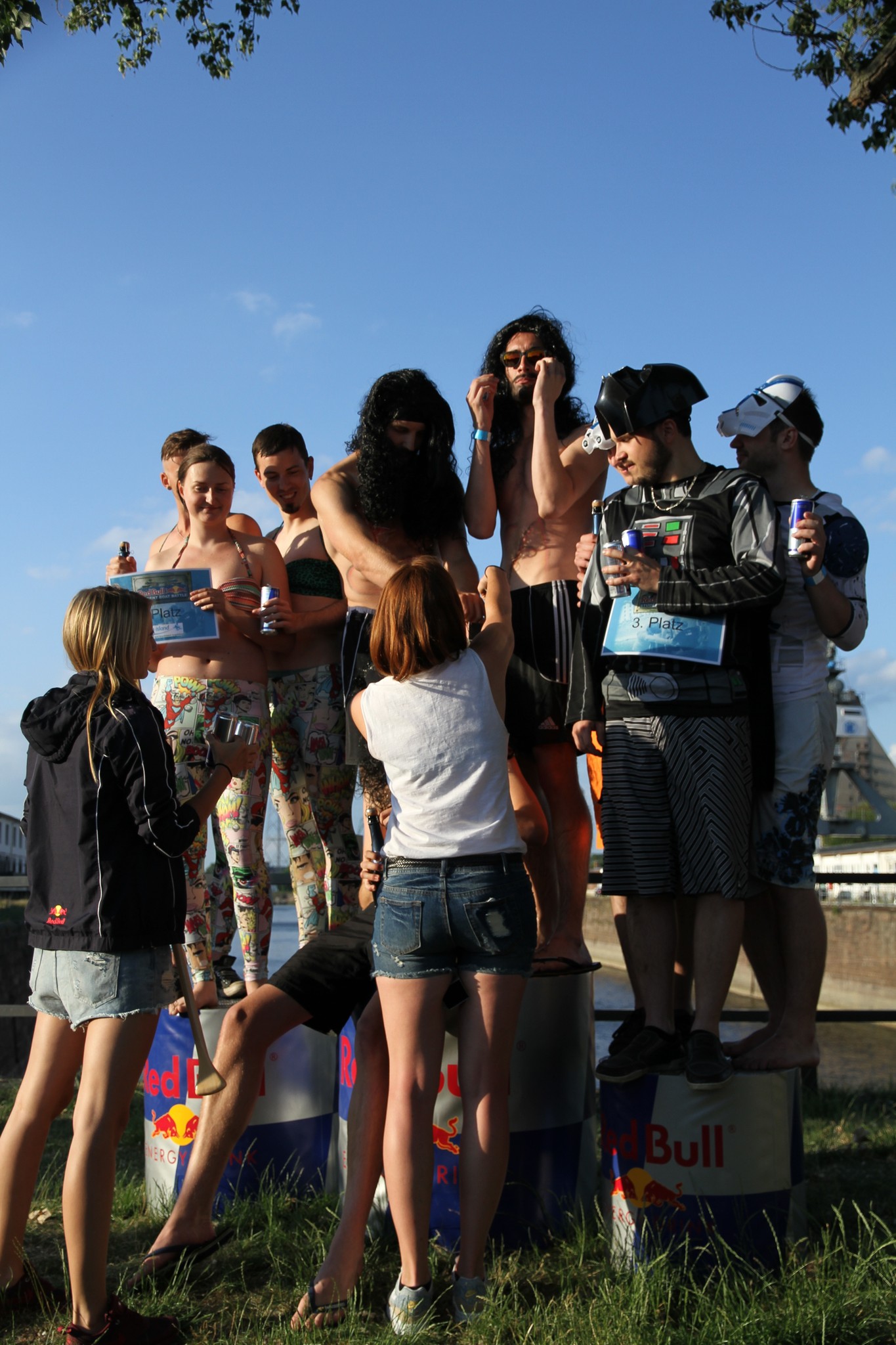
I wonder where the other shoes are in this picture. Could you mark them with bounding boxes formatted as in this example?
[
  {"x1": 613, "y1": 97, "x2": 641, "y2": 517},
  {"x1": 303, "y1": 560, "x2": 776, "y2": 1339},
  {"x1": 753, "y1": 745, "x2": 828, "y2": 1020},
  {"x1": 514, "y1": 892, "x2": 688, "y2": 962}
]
[
  {"x1": 595, "y1": 1026, "x2": 681, "y2": 1084},
  {"x1": 673, "y1": 1006, "x2": 694, "y2": 1034},
  {"x1": 389, "y1": 1267, "x2": 442, "y2": 1334},
  {"x1": 607, "y1": 1006, "x2": 646, "y2": 1055},
  {"x1": 684, "y1": 1029, "x2": 733, "y2": 1090},
  {"x1": 448, "y1": 1272, "x2": 489, "y2": 1321},
  {"x1": 0, "y1": 1259, "x2": 69, "y2": 1317},
  {"x1": 212, "y1": 955, "x2": 246, "y2": 998},
  {"x1": 57, "y1": 1297, "x2": 181, "y2": 1344}
]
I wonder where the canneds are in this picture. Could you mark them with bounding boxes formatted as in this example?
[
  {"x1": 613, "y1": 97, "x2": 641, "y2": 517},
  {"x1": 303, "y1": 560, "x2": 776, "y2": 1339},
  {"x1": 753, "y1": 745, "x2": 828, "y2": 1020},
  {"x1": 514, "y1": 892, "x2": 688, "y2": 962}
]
[
  {"x1": 206, "y1": 710, "x2": 238, "y2": 770},
  {"x1": 602, "y1": 539, "x2": 631, "y2": 599},
  {"x1": 621, "y1": 529, "x2": 644, "y2": 586},
  {"x1": 231, "y1": 720, "x2": 260, "y2": 780},
  {"x1": 260, "y1": 584, "x2": 280, "y2": 635},
  {"x1": 788, "y1": 499, "x2": 815, "y2": 558}
]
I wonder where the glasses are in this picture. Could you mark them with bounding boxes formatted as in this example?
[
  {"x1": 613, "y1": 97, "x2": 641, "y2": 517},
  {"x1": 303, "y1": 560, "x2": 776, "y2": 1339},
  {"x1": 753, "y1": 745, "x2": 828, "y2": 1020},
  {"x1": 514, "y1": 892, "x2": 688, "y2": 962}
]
[{"x1": 500, "y1": 347, "x2": 547, "y2": 367}]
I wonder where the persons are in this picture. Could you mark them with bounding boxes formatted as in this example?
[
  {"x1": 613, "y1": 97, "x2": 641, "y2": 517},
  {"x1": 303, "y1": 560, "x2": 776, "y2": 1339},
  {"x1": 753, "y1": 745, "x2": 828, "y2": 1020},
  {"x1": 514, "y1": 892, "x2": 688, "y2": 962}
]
[
  {"x1": 0, "y1": 585, "x2": 263, "y2": 1344},
  {"x1": 106, "y1": 305, "x2": 870, "y2": 1335}
]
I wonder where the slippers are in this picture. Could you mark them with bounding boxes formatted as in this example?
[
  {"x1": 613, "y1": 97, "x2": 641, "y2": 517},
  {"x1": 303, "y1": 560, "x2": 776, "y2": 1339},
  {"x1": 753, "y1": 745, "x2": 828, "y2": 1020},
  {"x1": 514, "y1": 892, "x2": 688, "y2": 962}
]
[
  {"x1": 529, "y1": 958, "x2": 601, "y2": 977},
  {"x1": 129, "y1": 1227, "x2": 234, "y2": 1291},
  {"x1": 292, "y1": 1275, "x2": 361, "y2": 1328}
]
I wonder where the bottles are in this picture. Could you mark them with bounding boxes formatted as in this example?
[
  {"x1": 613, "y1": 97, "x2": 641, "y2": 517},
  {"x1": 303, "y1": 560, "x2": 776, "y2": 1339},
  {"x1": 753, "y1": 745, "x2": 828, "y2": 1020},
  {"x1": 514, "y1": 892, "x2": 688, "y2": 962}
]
[{"x1": 118, "y1": 542, "x2": 131, "y2": 557}]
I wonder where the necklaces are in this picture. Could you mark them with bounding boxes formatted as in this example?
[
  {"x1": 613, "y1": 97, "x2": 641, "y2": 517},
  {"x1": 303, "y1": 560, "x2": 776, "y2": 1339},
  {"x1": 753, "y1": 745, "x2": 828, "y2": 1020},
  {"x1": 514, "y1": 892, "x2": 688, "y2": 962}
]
[{"x1": 176, "y1": 525, "x2": 185, "y2": 539}]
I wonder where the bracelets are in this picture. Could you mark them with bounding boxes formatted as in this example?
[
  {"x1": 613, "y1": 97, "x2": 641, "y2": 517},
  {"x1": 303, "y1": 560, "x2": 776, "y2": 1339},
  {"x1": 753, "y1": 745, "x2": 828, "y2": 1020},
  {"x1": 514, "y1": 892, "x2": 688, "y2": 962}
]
[
  {"x1": 214, "y1": 763, "x2": 233, "y2": 779},
  {"x1": 803, "y1": 565, "x2": 827, "y2": 585},
  {"x1": 484, "y1": 565, "x2": 507, "y2": 578},
  {"x1": 472, "y1": 430, "x2": 491, "y2": 442}
]
